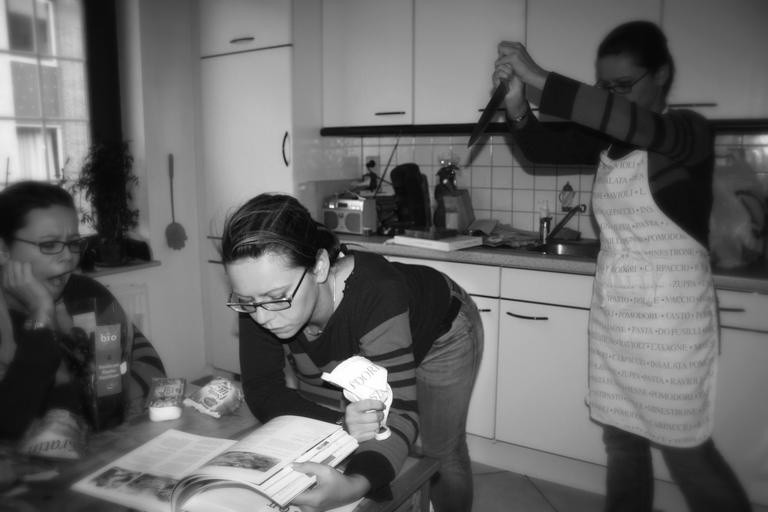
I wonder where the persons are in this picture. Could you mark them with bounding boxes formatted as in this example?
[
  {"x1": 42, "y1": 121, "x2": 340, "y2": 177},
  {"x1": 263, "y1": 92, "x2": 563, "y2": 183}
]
[
  {"x1": 492, "y1": 20, "x2": 752, "y2": 511},
  {"x1": 0, "y1": 181, "x2": 168, "y2": 443},
  {"x1": 215, "y1": 192, "x2": 485, "y2": 510}
]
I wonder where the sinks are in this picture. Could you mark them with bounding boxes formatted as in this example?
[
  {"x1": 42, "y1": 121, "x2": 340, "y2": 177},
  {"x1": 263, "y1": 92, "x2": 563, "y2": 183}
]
[{"x1": 517, "y1": 239, "x2": 601, "y2": 262}]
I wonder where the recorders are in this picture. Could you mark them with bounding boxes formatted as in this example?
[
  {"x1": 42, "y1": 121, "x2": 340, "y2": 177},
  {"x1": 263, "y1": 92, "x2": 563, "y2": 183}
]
[{"x1": 322, "y1": 196, "x2": 376, "y2": 234}]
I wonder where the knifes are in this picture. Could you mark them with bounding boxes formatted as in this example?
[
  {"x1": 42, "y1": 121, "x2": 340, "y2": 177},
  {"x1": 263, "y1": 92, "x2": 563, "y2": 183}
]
[{"x1": 465, "y1": 67, "x2": 515, "y2": 147}]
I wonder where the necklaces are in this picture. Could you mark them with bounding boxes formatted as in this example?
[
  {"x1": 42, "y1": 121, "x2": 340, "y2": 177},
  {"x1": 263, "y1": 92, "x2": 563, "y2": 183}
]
[{"x1": 304, "y1": 271, "x2": 337, "y2": 338}]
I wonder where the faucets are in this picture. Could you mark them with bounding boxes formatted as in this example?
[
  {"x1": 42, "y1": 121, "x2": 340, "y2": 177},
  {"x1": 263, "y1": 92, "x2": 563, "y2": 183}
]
[{"x1": 539, "y1": 204, "x2": 587, "y2": 244}]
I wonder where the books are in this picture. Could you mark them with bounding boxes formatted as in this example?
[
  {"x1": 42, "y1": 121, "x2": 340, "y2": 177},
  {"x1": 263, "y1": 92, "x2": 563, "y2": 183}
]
[{"x1": 68, "y1": 415, "x2": 364, "y2": 512}]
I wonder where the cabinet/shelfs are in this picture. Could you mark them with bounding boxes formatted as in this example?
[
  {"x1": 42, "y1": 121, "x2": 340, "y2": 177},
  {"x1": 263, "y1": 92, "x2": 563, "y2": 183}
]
[
  {"x1": 664, "y1": 1, "x2": 768, "y2": 135},
  {"x1": 198, "y1": 0, "x2": 325, "y2": 60},
  {"x1": 322, "y1": 0, "x2": 414, "y2": 135},
  {"x1": 383, "y1": 242, "x2": 609, "y2": 497},
  {"x1": 415, "y1": 0, "x2": 527, "y2": 136},
  {"x1": 649, "y1": 276, "x2": 767, "y2": 511},
  {"x1": 206, "y1": 260, "x2": 242, "y2": 377},
  {"x1": 526, "y1": 0, "x2": 664, "y2": 133},
  {"x1": 198, "y1": 44, "x2": 325, "y2": 240}
]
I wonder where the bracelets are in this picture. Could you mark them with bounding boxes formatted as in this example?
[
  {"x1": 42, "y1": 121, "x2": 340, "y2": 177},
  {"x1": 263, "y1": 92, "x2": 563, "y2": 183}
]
[
  {"x1": 507, "y1": 104, "x2": 527, "y2": 120},
  {"x1": 24, "y1": 316, "x2": 54, "y2": 335}
]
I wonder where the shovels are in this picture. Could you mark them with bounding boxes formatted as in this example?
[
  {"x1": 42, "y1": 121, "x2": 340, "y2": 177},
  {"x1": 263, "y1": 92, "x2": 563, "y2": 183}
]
[{"x1": 164, "y1": 153, "x2": 187, "y2": 251}]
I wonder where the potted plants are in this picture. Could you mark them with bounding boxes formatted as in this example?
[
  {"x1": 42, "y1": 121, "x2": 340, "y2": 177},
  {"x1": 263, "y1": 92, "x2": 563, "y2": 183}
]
[{"x1": 66, "y1": 138, "x2": 140, "y2": 267}]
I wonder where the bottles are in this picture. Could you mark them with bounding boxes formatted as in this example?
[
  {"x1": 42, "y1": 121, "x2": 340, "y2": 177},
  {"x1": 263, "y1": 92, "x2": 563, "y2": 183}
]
[{"x1": 539, "y1": 198, "x2": 550, "y2": 245}]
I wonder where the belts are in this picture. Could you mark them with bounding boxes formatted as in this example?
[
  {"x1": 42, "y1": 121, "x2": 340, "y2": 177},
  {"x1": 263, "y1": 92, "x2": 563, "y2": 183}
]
[{"x1": 435, "y1": 279, "x2": 460, "y2": 338}]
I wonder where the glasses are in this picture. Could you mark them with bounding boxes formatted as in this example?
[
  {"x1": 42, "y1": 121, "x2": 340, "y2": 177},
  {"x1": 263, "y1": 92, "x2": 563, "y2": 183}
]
[
  {"x1": 16, "y1": 237, "x2": 90, "y2": 255},
  {"x1": 594, "y1": 69, "x2": 649, "y2": 94},
  {"x1": 226, "y1": 269, "x2": 308, "y2": 314}
]
